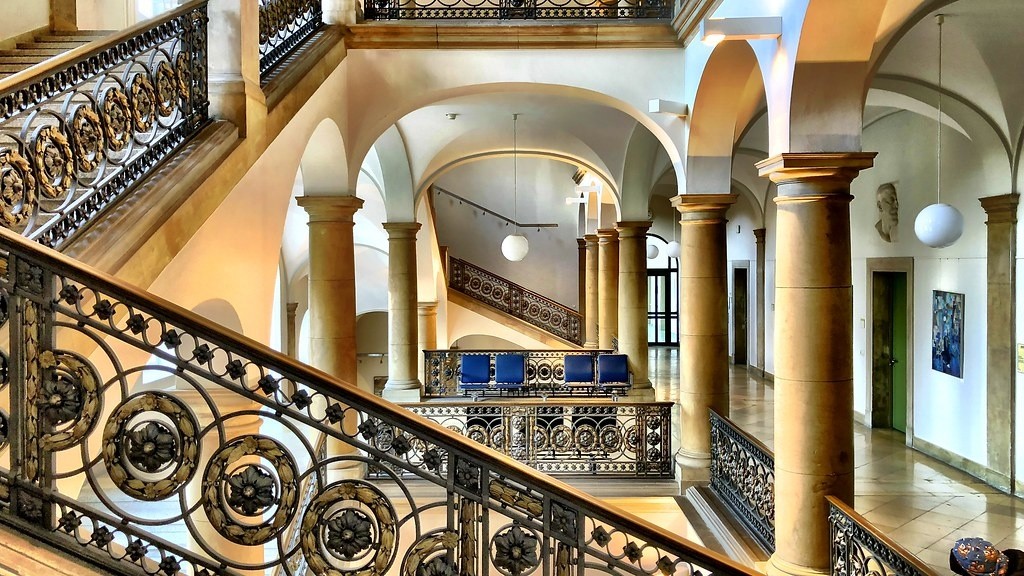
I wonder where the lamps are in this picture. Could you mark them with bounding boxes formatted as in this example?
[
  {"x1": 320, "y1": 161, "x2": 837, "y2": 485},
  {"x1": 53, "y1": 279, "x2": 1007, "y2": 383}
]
[
  {"x1": 501, "y1": 112, "x2": 530, "y2": 262},
  {"x1": 914, "y1": 12, "x2": 963, "y2": 247},
  {"x1": 666, "y1": 208, "x2": 680, "y2": 257},
  {"x1": 647, "y1": 232, "x2": 658, "y2": 259}
]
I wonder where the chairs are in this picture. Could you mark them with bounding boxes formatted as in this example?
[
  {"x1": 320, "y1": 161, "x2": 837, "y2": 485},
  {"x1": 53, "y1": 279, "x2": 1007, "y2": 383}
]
[
  {"x1": 564, "y1": 355, "x2": 596, "y2": 396},
  {"x1": 598, "y1": 354, "x2": 630, "y2": 398},
  {"x1": 494, "y1": 354, "x2": 524, "y2": 396},
  {"x1": 459, "y1": 355, "x2": 491, "y2": 397}
]
